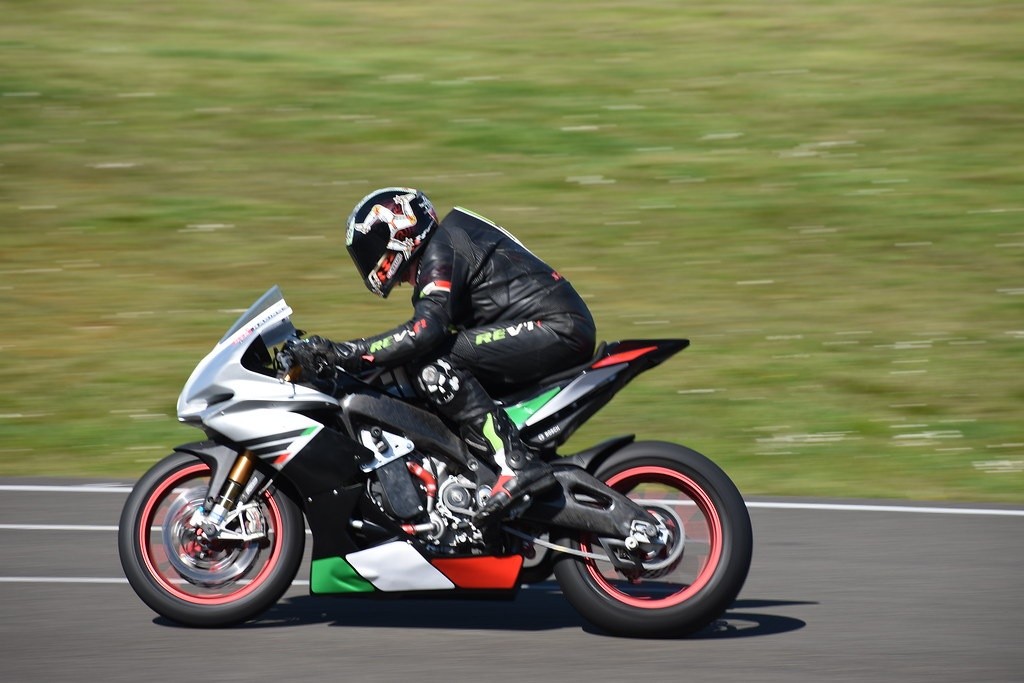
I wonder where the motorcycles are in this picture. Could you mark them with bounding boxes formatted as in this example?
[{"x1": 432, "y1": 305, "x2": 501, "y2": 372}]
[{"x1": 115, "y1": 283, "x2": 753, "y2": 639}]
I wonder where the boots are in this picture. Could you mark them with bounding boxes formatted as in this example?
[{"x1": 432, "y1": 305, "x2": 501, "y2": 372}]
[{"x1": 457, "y1": 407, "x2": 557, "y2": 527}]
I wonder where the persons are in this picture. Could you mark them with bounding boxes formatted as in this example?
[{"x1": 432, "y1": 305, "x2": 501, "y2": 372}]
[{"x1": 289, "y1": 186, "x2": 596, "y2": 524}]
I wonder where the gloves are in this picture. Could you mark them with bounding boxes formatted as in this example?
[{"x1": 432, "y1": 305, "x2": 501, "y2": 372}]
[{"x1": 289, "y1": 334, "x2": 337, "y2": 378}]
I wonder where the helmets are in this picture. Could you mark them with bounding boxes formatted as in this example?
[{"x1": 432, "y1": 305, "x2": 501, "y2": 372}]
[{"x1": 346, "y1": 186, "x2": 437, "y2": 299}]
